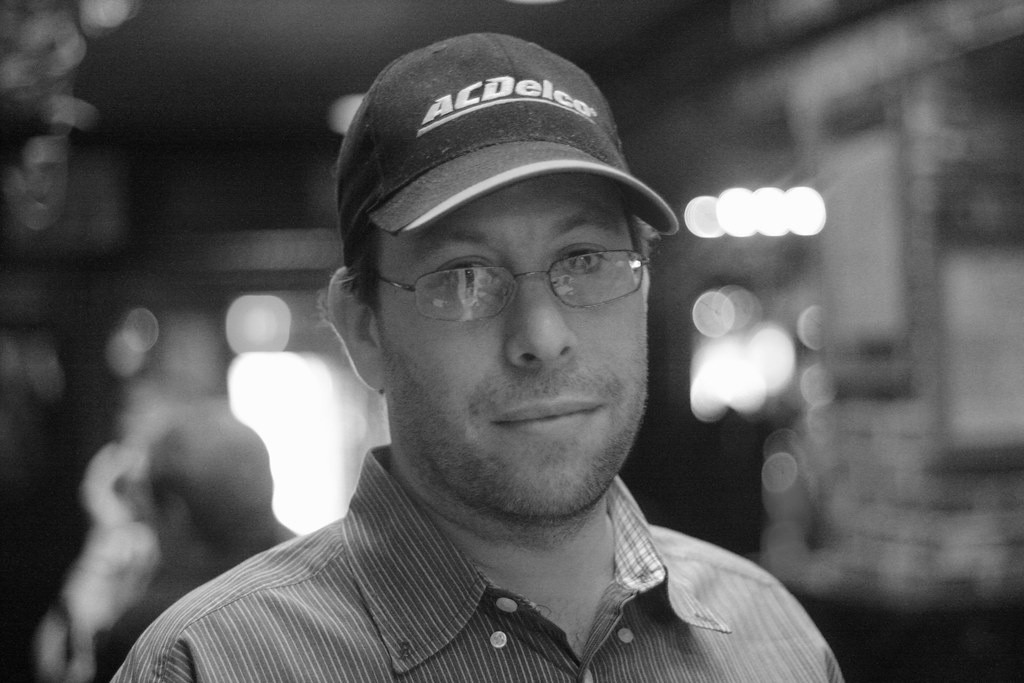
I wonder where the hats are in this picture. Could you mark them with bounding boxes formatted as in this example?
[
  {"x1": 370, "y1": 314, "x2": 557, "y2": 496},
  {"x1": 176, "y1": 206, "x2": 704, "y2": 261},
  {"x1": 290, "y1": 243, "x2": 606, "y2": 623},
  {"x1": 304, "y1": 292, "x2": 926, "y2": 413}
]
[{"x1": 339, "y1": 32, "x2": 679, "y2": 275}]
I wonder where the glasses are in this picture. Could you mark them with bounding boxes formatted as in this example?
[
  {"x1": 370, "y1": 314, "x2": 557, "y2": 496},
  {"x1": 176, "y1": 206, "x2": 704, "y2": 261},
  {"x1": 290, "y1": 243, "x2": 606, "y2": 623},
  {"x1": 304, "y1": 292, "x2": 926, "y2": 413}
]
[{"x1": 353, "y1": 249, "x2": 650, "y2": 322}]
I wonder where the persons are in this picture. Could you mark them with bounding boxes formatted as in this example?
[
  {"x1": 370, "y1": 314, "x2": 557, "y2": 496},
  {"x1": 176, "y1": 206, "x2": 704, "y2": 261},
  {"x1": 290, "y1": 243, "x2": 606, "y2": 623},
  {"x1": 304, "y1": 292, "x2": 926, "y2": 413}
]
[{"x1": 107, "y1": 28, "x2": 848, "y2": 683}]
